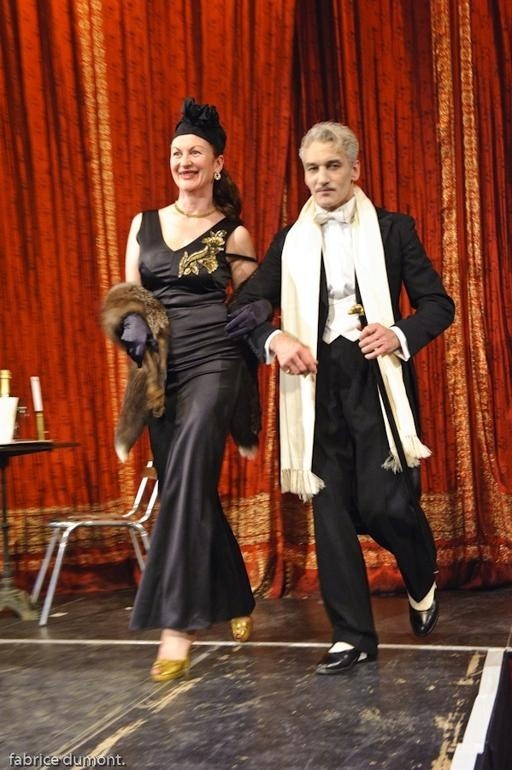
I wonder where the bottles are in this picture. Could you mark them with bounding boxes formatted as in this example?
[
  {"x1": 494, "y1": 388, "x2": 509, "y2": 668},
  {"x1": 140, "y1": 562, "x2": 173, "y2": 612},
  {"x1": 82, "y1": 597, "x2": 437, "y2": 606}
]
[{"x1": 0, "y1": 369, "x2": 34, "y2": 444}]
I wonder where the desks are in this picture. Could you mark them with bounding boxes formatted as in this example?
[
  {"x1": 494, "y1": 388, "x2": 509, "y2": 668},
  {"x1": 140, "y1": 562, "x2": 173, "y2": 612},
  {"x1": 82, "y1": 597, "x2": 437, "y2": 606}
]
[{"x1": 0, "y1": 439, "x2": 54, "y2": 622}]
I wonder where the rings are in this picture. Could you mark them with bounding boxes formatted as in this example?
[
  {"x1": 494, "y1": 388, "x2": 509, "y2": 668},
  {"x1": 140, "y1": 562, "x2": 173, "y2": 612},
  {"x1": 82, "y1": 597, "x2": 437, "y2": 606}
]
[{"x1": 284, "y1": 367, "x2": 291, "y2": 375}]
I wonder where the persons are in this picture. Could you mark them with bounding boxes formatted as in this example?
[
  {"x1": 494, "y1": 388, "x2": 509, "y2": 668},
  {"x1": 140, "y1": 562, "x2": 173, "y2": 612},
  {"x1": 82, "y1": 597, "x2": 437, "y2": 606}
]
[
  {"x1": 226, "y1": 119, "x2": 457, "y2": 677},
  {"x1": 117, "y1": 96, "x2": 271, "y2": 681}
]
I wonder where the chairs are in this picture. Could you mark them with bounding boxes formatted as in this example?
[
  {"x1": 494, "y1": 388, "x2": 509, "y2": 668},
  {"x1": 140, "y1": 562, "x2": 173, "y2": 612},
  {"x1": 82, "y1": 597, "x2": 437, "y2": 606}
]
[{"x1": 29, "y1": 446, "x2": 181, "y2": 628}]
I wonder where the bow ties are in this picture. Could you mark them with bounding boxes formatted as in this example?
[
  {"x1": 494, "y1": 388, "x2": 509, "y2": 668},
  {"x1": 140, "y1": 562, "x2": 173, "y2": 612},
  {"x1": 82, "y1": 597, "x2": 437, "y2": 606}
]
[{"x1": 313, "y1": 210, "x2": 345, "y2": 224}]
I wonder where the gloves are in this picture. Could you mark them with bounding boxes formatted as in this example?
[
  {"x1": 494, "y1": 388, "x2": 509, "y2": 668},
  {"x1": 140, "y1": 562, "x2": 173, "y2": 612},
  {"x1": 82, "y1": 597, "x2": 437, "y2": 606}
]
[
  {"x1": 120, "y1": 314, "x2": 161, "y2": 368},
  {"x1": 225, "y1": 299, "x2": 272, "y2": 339}
]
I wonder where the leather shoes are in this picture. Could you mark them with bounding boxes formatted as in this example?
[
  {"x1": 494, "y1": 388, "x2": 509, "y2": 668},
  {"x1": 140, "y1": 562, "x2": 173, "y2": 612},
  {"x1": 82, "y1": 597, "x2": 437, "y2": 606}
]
[
  {"x1": 316, "y1": 639, "x2": 369, "y2": 675},
  {"x1": 406, "y1": 583, "x2": 439, "y2": 637},
  {"x1": 149, "y1": 628, "x2": 196, "y2": 681},
  {"x1": 230, "y1": 617, "x2": 254, "y2": 642}
]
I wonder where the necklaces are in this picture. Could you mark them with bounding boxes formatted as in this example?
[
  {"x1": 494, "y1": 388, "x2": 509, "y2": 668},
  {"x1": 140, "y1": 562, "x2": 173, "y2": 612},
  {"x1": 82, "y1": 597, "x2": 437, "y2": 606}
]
[{"x1": 173, "y1": 200, "x2": 218, "y2": 218}]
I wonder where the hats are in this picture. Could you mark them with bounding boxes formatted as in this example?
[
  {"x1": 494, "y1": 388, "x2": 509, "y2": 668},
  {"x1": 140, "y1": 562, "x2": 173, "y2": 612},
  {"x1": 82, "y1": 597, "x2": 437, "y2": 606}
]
[{"x1": 171, "y1": 96, "x2": 227, "y2": 152}]
